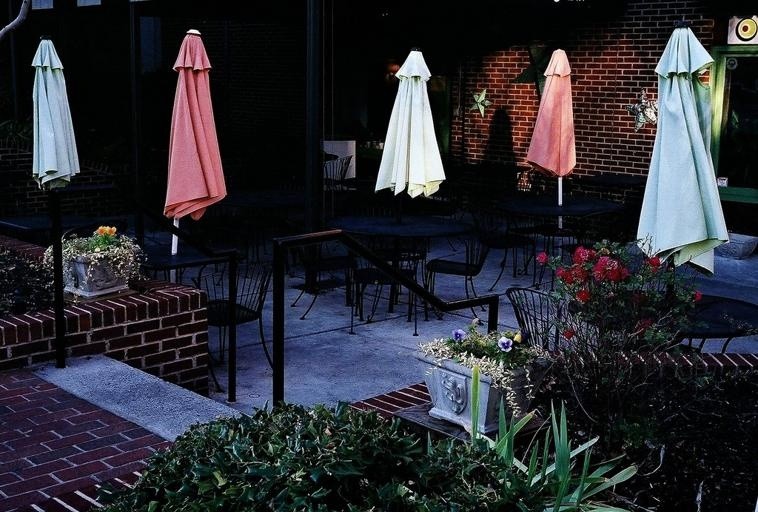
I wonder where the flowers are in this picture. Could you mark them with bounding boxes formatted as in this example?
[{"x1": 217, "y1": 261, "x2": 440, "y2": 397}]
[
  {"x1": 415, "y1": 317, "x2": 556, "y2": 421},
  {"x1": 533, "y1": 234, "x2": 704, "y2": 362},
  {"x1": 42, "y1": 225, "x2": 151, "y2": 307}
]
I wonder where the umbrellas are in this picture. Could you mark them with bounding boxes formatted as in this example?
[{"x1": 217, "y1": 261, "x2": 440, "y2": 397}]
[
  {"x1": 31, "y1": 33, "x2": 81, "y2": 229},
  {"x1": 374, "y1": 48, "x2": 448, "y2": 257},
  {"x1": 161, "y1": 28, "x2": 228, "y2": 284},
  {"x1": 636, "y1": 14, "x2": 730, "y2": 351},
  {"x1": 527, "y1": 48, "x2": 577, "y2": 258}
]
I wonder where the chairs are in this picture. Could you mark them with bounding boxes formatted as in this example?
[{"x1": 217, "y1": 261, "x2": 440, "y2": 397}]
[{"x1": 194, "y1": 198, "x2": 628, "y2": 394}]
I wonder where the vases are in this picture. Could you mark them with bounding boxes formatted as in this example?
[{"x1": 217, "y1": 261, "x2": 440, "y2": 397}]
[
  {"x1": 64, "y1": 248, "x2": 131, "y2": 300},
  {"x1": 413, "y1": 349, "x2": 552, "y2": 435}
]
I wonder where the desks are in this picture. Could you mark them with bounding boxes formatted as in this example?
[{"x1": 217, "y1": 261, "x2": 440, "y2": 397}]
[{"x1": 136, "y1": 244, "x2": 248, "y2": 283}]
